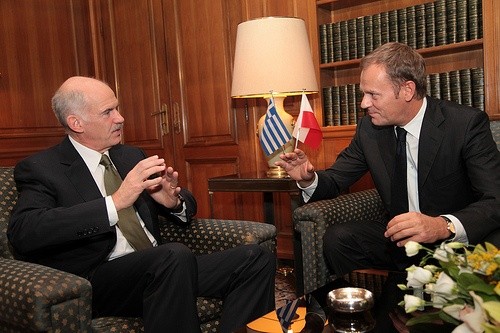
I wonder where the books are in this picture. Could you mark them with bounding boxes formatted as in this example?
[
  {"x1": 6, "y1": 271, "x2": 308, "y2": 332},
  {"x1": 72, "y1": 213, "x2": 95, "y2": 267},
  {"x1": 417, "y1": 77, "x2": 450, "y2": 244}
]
[
  {"x1": 323, "y1": 67, "x2": 484, "y2": 127},
  {"x1": 319, "y1": 0, "x2": 482, "y2": 64}
]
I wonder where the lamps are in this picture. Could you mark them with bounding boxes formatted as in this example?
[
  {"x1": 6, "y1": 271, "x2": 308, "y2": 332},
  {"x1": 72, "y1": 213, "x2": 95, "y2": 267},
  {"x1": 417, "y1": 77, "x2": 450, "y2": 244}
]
[{"x1": 231, "y1": 16, "x2": 319, "y2": 179}]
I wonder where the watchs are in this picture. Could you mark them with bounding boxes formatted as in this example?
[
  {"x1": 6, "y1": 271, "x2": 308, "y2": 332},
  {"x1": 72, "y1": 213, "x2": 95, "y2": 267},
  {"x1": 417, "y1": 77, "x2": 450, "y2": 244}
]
[{"x1": 442, "y1": 216, "x2": 455, "y2": 239}]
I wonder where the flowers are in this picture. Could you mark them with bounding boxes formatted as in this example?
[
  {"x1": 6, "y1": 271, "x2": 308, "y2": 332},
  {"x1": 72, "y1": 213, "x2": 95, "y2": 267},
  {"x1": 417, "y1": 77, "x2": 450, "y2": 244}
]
[{"x1": 397, "y1": 238, "x2": 500, "y2": 333}]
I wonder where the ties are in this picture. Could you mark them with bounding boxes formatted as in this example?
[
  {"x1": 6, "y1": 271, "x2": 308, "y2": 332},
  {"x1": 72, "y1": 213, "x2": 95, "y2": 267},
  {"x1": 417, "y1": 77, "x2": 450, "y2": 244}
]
[
  {"x1": 99, "y1": 154, "x2": 153, "y2": 252},
  {"x1": 393, "y1": 126, "x2": 409, "y2": 215}
]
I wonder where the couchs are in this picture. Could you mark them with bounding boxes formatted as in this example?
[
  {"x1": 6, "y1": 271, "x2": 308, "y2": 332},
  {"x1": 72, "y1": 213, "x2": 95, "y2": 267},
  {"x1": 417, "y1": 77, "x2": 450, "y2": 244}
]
[
  {"x1": 0, "y1": 166, "x2": 278, "y2": 333},
  {"x1": 292, "y1": 120, "x2": 500, "y2": 293}
]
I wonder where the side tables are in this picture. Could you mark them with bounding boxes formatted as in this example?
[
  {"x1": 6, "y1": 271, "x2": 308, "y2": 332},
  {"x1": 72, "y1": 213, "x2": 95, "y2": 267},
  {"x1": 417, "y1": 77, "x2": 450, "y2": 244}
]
[{"x1": 208, "y1": 173, "x2": 316, "y2": 298}]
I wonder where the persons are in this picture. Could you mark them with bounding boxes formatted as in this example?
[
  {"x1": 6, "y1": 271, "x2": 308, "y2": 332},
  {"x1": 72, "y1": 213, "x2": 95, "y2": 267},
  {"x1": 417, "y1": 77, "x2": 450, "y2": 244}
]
[
  {"x1": 300, "y1": 279, "x2": 463, "y2": 333},
  {"x1": 274, "y1": 42, "x2": 500, "y2": 277},
  {"x1": 6, "y1": 75, "x2": 277, "y2": 333}
]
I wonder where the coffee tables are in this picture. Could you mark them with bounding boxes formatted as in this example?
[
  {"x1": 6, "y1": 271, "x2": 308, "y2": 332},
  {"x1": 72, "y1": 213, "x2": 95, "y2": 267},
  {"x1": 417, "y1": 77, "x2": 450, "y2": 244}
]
[{"x1": 250, "y1": 269, "x2": 443, "y2": 333}]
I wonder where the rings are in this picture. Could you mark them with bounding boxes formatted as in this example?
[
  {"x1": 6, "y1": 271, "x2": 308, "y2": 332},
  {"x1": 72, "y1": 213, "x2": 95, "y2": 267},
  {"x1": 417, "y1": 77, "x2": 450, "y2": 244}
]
[{"x1": 171, "y1": 186, "x2": 175, "y2": 189}]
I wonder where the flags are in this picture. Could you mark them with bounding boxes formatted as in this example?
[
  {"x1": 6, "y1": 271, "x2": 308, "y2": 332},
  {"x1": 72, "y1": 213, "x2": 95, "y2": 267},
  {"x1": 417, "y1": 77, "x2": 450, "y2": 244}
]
[
  {"x1": 292, "y1": 93, "x2": 322, "y2": 151},
  {"x1": 260, "y1": 99, "x2": 292, "y2": 156},
  {"x1": 276, "y1": 299, "x2": 301, "y2": 333}
]
